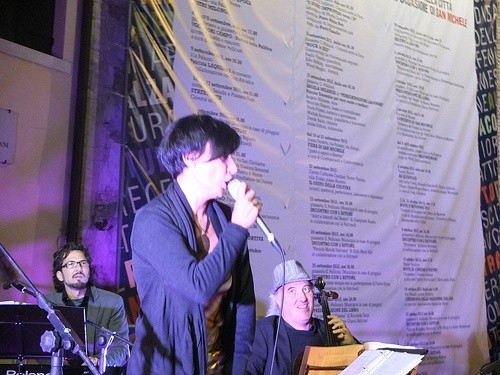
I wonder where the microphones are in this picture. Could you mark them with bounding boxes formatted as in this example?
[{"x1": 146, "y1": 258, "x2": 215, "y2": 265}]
[{"x1": 228, "y1": 179, "x2": 283, "y2": 250}]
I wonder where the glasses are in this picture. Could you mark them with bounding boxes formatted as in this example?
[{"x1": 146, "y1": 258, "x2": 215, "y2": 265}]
[
  {"x1": 197, "y1": 228, "x2": 210, "y2": 258},
  {"x1": 61, "y1": 259, "x2": 90, "y2": 269}
]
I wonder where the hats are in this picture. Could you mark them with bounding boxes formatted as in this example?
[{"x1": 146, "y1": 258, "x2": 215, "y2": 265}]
[{"x1": 273, "y1": 259, "x2": 313, "y2": 292}]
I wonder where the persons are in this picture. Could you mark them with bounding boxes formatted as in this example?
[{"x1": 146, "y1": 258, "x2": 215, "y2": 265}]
[
  {"x1": 245, "y1": 259, "x2": 364, "y2": 375},
  {"x1": 46, "y1": 242, "x2": 130, "y2": 367},
  {"x1": 126, "y1": 113, "x2": 262, "y2": 375}
]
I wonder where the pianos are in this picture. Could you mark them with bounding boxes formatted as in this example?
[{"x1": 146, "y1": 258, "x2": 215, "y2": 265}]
[{"x1": 0, "y1": 302, "x2": 127, "y2": 375}]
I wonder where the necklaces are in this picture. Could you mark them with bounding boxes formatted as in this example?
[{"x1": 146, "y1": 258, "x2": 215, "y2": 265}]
[{"x1": 194, "y1": 216, "x2": 211, "y2": 256}]
